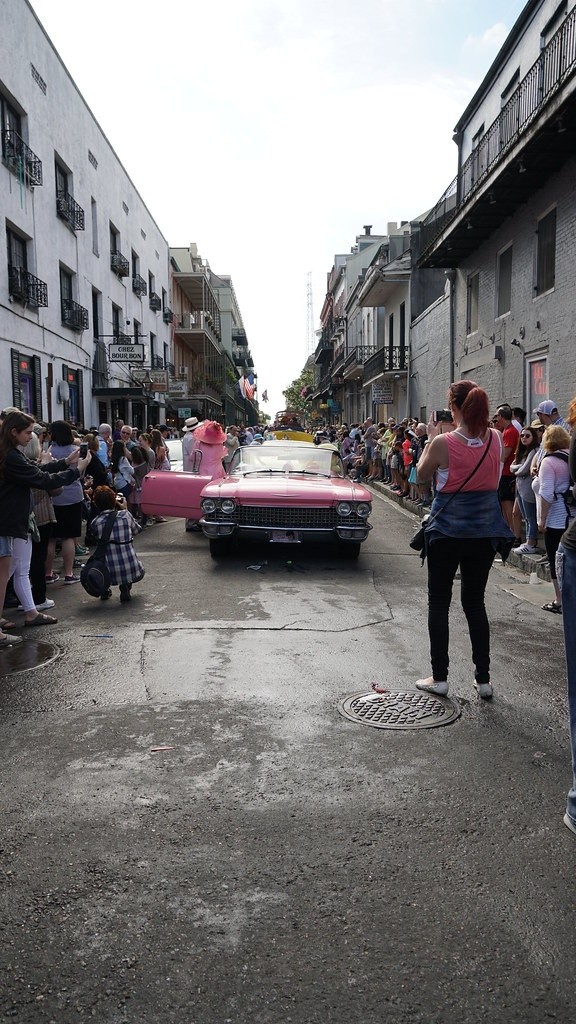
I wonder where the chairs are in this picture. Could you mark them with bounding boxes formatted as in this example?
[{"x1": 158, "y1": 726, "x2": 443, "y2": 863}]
[
  {"x1": 305, "y1": 461, "x2": 319, "y2": 470},
  {"x1": 240, "y1": 452, "x2": 272, "y2": 471}
]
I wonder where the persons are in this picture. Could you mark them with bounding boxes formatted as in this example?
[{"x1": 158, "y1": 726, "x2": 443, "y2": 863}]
[
  {"x1": 181, "y1": 417, "x2": 203, "y2": 532},
  {"x1": 220, "y1": 423, "x2": 274, "y2": 474},
  {"x1": 167, "y1": 426, "x2": 186, "y2": 440},
  {"x1": 288, "y1": 417, "x2": 299, "y2": 427},
  {"x1": 0, "y1": 406, "x2": 171, "y2": 647},
  {"x1": 487, "y1": 397, "x2": 576, "y2": 834},
  {"x1": 189, "y1": 420, "x2": 228, "y2": 481},
  {"x1": 84, "y1": 484, "x2": 146, "y2": 604},
  {"x1": 304, "y1": 416, "x2": 436, "y2": 511},
  {"x1": 414, "y1": 380, "x2": 521, "y2": 696}
]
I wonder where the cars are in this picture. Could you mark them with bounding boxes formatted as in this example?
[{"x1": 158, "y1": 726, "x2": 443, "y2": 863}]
[
  {"x1": 166, "y1": 439, "x2": 186, "y2": 474},
  {"x1": 140, "y1": 440, "x2": 375, "y2": 561}
]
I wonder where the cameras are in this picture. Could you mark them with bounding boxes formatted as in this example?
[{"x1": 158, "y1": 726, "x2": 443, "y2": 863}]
[{"x1": 115, "y1": 492, "x2": 123, "y2": 508}]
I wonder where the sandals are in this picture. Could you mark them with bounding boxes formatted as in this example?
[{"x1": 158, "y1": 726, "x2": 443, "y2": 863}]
[{"x1": 540, "y1": 600, "x2": 563, "y2": 615}]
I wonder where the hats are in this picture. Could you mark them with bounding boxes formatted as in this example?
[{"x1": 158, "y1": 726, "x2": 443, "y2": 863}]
[
  {"x1": 530, "y1": 419, "x2": 544, "y2": 429},
  {"x1": 33, "y1": 422, "x2": 47, "y2": 433},
  {"x1": 533, "y1": 399, "x2": 558, "y2": 416},
  {"x1": 254, "y1": 434, "x2": 264, "y2": 440},
  {"x1": 194, "y1": 421, "x2": 228, "y2": 445},
  {"x1": 182, "y1": 416, "x2": 203, "y2": 432}
]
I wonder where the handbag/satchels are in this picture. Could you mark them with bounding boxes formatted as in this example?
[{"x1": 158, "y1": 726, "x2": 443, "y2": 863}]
[
  {"x1": 410, "y1": 514, "x2": 428, "y2": 567},
  {"x1": 128, "y1": 482, "x2": 136, "y2": 491},
  {"x1": 80, "y1": 557, "x2": 110, "y2": 596}
]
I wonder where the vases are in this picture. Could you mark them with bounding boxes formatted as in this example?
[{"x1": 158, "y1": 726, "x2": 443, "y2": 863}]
[
  {"x1": 214, "y1": 330, "x2": 217, "y2": 335},
  {"x1": 205, "y1": 317, "x2": 210, "y2": 322},
  {"x1": 218, "y1": 338, "x2": 221, "y2": 343},
  {"x1": 211, "y1": 326, "x2": 214, "y2": 331},
  {"x1": 208, "y1": 322, "x2": 212, "y2": 326},
  {"x1": 216, "y1": 334, "x2": 219, "y2": 339}
]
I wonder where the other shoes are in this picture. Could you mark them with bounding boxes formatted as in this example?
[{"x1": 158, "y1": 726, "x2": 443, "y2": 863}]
[
  {"x1": 348, "y1": 472, "x2": 433, "y2": 507},
  {"x1": 72, "y1": 557, "x2": 84, "y2": 568},
  {"x1": 155, "y1": 517, "x2": 167, "y2": 522},
  {"x1": 120, "y1": 592, "x2": 131, "y2": 603},
  {"x1": 472, "y1": 678, "x2": 492, "y2": 697},
  {"x1": 23, "y1": 573, "x2": 81, "y2": 626},
  {"x1": 536, "y1": 551, "x2": 549, "y2": 565},
  {"x1": 415, "y1": 677, "x2": 448, "y2": 696},
  {"x1": 146, "y1": 518, "x2": 153, "y2": 526},
  {"x1": 101, "y1": 589, "x2": 112, "y2": 600},
  {"x1": 0, "y1": 632, "x2": 23, "y2": 648},
  {"x1": 74, "y1": 543, "x2": 90, "y2": 556}
]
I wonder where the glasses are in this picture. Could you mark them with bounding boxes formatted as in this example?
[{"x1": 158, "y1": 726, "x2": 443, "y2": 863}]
[
  {"x1": 492, "y1": 419, "x2": 500, "y2": 423},
  {"x1": 520, "y1": 434, "x2": 533, "y2": 438},
  {"x1": 534, "y1": 427, "x2": 543, "y2": 431},
  {"x1": 122, "y1": 432, "x2": 131, "y2": 435}
]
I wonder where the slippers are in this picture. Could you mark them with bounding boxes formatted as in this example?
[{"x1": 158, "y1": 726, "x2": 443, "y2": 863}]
[{"x1": 0, "y1": 618, "x2": 17, "y2": 628}]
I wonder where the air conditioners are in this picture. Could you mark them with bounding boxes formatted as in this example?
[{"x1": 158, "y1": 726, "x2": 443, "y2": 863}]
[
  {"x1": 59, "y1": 198, "x2": 68, "y2": 220},
  {"x1": 133, "y1": 278, "x2": 140, "y2": 290},
  {"x1": 180, "y1": 366, "x2": 188, "y2": 374},
  {"x1": 111, "y1": 255, "x2": 123, "y2": 270}
]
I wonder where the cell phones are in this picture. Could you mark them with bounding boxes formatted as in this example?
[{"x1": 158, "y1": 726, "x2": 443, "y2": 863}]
[
  {"x1": 433, "y1": 411, "x2": 454, "y2": 423},
  {"x1": 79, "y1": 443, "x2": 88, "y2": 460}
]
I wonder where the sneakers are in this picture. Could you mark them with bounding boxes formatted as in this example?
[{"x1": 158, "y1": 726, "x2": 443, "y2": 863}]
[{"x1": 514, "y1": 544, "x2": 536, "y2": 554}]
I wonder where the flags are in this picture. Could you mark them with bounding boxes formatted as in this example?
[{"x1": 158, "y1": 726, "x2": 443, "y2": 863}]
[
  {"x1": 244, "y1": 375, "x2": 254, "y2": 400},
  {"x1": 238, "y1": 376, "x2": 246, "y2": 399}
]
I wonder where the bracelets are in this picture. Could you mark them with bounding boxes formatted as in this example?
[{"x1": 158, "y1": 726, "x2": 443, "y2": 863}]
[{"x1": 424, "y1": 440, "x2": 431, "y2": 444}]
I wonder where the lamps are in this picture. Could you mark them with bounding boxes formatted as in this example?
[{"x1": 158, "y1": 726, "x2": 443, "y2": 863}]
[
  {"x1": 516, "y1": 159, "x2": 526, "y2": 173},
  {"x1": 512, "y1": 339, "x2": 520, "y2": 347},
  {"x1": 446, "y1": 241, "x2": 452, "y2": 251},
  {"x1": 489, "y1": 193, "x2": 497, "y2": 205},
  {"x1": 56, "y1": 377, "x2": 70, "y2": 404},
  {"x1": 556, "y1": 118, "x2": 566, "y2": 133},
  {"x1": 465, "y1": 218, "x2": 473, "y2": 229}
]
